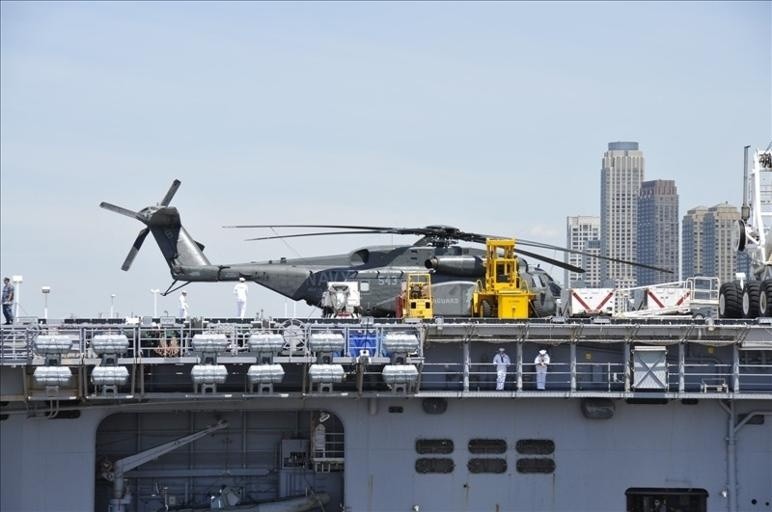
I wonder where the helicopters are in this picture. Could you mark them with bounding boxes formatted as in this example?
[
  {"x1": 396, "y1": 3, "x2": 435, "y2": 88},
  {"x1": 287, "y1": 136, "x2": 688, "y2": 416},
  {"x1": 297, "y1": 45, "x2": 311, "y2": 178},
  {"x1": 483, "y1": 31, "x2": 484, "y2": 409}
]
[{"x1": 99, "y1": 179, "x2": 675, "y2": 316}]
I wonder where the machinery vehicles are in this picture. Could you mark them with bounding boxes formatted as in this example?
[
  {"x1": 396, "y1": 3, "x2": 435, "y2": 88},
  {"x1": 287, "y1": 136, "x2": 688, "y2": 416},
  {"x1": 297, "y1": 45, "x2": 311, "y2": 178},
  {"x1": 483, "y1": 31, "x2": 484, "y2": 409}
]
[
  {"x1": 472, "y1": 238, "x2": 536, "y2": 319},
  {"x1": 401, "y1": 274, "x2": 433, "y2": 318}
]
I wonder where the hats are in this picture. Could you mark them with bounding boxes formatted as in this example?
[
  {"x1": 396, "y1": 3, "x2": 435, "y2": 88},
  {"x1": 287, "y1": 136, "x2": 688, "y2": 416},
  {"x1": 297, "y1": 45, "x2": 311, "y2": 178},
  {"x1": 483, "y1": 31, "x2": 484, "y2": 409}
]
[
  {"x1": 240, "y1": 278, "x2": 245, "y2": 281},
  {"x1": 499, "y1": 348, "x2": 505, "y2": 351},
  {"x1": 539, "y1": 350, "x2": 546, "y2": 355},
  {"x1": 182, "y1": 291, "x2": 187, "y2": 293}
]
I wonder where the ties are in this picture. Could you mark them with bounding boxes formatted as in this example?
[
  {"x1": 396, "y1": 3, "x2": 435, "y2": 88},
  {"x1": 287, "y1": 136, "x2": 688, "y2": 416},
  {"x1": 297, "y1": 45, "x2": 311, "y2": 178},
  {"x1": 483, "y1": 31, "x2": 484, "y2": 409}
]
[{"x1": 500, "y1": 354, "x2": 504, "y2": 363}]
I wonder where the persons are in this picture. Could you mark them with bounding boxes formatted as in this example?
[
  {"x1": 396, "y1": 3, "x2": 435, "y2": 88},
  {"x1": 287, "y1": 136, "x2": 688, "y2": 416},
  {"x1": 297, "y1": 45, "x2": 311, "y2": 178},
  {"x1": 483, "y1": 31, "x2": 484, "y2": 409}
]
[
  {"x1": 1, "y1": 277, "x2": 15, "y2": 324},
  {"x1": 534, "y1": 348, "x2": 550, "y2": 391},
  {"x1": 493, "y1": 347, "x2": 511, "y2": 390},
  {"x1": 233, "y1": 277, "x2": 248, "y2": 317},
  {"x1": 178, "y1": 290, "x2": 188, "y2": 318}
]
